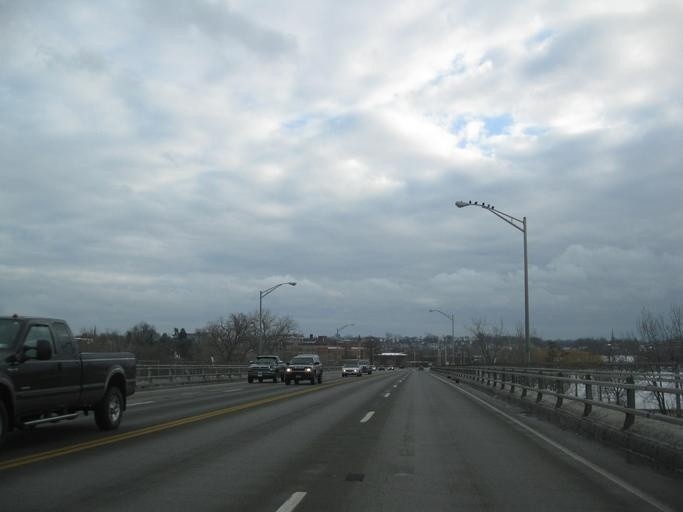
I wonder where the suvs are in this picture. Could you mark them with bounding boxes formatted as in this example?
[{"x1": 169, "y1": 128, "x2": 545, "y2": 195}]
[
  {"x1": 284, "y1": 354, "x2": 324, "y2": 386},
  {"x1": 246, "y1": 355, "x2": 286, "y2": 384},
  {"x1": 357, "y1": 360, "x2": 373, "y2": 375}
]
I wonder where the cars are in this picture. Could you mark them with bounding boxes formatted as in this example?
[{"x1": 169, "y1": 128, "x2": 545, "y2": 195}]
[
  {"x1": 341, "y1": 362, "x2": 361, "y2": 377},
  {"x1": 386, "y1": 366, "x2": 394, "y2": 371},
  {"x1": 377, "y1": 366, "x2": 385, "y2": 371},
  {"x1": 370, "y1": 364, "x2": 377, "y2": 371}
]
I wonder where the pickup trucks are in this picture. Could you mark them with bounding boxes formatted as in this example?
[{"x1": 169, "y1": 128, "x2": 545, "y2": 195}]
[{"x1": 0, "y1": 315, "x2": 136, "y2": 442}]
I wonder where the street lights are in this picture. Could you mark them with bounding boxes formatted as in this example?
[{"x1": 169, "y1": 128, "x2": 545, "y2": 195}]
[
  {"x1": 258, "y1": 280, "x2": 296, "y2": 353},
  {"x1": 453, "y1": 199, "x2": 530, "y2": 367},
  {"x1": 428, "y1": 309, "x2": 454, "y2": 365},
  {"x1": 335, "y1": 323, "x2": 355, "y2": 365}
]
